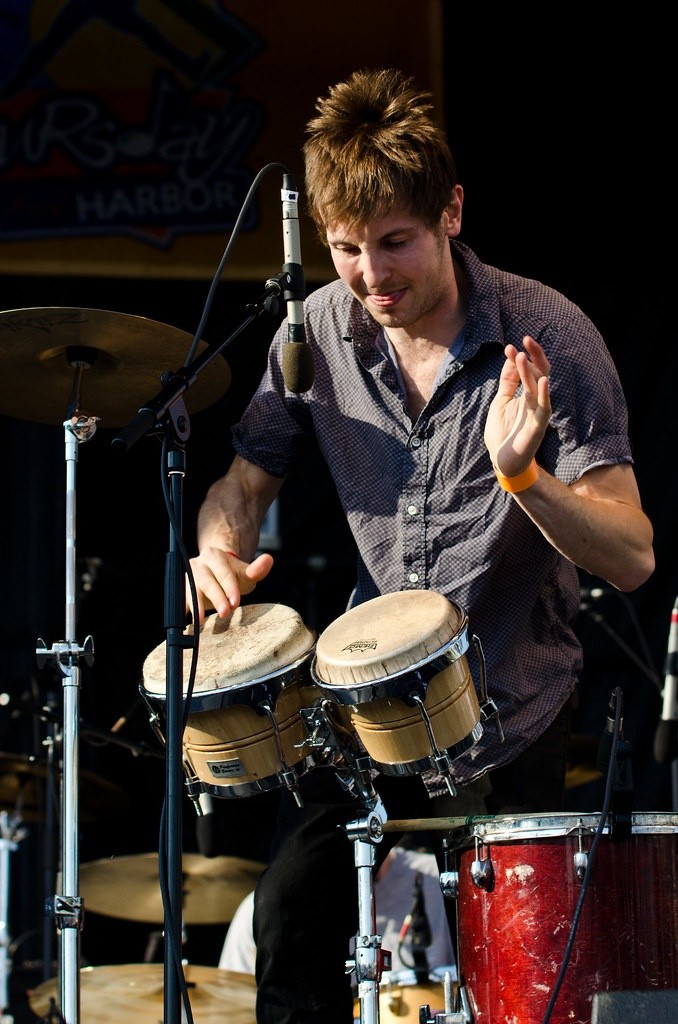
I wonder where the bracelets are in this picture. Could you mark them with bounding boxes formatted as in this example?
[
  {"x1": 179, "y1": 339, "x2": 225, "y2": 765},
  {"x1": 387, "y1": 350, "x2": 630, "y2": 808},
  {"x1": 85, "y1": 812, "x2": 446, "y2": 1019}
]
[{"x1": 495, "y1": 456, "x2": 540, "y2": 495}]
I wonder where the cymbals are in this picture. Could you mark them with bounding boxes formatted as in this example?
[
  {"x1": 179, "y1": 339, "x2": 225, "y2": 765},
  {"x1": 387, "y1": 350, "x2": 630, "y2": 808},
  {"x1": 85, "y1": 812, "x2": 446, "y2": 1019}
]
[
  {"x1": 78, "y1": 852, "x2": 270, "y2": 922},
  {"x1": 0, "y1": 751, "x2": 124, "y2": 822},
  {"x1": 27, "y1": 963, "x2": 256, "y2": 1023},
  {"x1": 0, "y1": 305, "x2": 233, "y2": 426}
]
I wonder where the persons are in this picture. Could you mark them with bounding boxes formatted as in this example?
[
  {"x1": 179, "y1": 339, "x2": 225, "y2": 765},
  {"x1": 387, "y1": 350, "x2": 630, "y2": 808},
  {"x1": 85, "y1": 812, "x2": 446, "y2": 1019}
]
[
  {"x1": 216, "y1": 845, "x2": 459, "y2": 989},
  {"x1": 185, "y1": 72, "x2": 656, "y2": 1024}
]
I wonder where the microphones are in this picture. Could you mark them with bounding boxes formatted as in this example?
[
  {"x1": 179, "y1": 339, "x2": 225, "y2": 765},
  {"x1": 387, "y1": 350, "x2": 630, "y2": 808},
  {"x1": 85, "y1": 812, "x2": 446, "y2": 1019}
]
[
  {"x1": 599, "y1": 688, "x2": 624, "y2": 771},
  {"x1": 654, "y1": 596, "x2": 678, "y2": 762},
  {"x1": 281, "y1": 173, "x2": 314, "y2": 394},
  {"x1": 410, "y1": 885, "x2": 432, "y2": 951}
]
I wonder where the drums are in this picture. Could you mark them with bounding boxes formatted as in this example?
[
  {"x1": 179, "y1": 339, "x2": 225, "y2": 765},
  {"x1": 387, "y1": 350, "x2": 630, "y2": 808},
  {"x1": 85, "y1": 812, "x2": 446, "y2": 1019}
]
[
  {"x1": 438, "y1": 809, "x2": 678, "y2": 1024},
  {"x1": 143, "y1": 602, "x2": 314, "y2": 799},
  {"x1": 311, "y1": 590, "x2": 485, "y2": 776}
]
